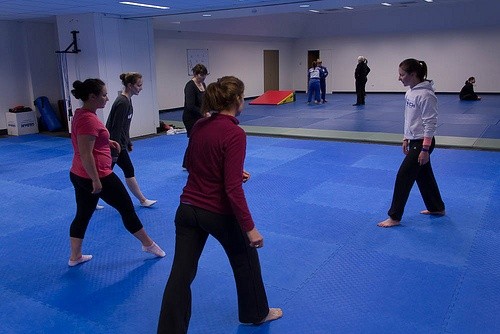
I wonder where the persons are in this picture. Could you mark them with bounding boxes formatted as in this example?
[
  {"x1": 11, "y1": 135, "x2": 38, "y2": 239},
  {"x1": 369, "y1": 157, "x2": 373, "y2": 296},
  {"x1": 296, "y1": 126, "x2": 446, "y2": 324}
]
[
  {"x1": 459, "y1": 76, "x2": 481, "y2": 100},
  {"x1": 182, "y1": 63, "x2": 212, "y2": 168},
  {"x1": 352, "y1": 56, "x2": 370, "y2": 106},
  {"x1": 377, "y1": 58, "x2": 445, "y2": 229},
  {"x1": 306, "y1": 59, "x2": 328, "y2": 105},
  {"x1": 157, "y1": 119, "x2": 173, "y2": 133},
  {"x1": 156, "y1": 75, "x2": 283, "y2": 334},
  {"x1": 96, "y1": 71, "x2": 158, "y2": 210},
  {"x1": 68, "y1": 77, "x2": 166, "y2": 267}
]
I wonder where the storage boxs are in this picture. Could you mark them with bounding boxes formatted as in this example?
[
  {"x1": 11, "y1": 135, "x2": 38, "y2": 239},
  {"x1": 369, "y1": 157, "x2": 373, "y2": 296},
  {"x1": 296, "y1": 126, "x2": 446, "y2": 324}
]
[{"x1": 6, "y1": 112, "x2": 39, "y2": 136}]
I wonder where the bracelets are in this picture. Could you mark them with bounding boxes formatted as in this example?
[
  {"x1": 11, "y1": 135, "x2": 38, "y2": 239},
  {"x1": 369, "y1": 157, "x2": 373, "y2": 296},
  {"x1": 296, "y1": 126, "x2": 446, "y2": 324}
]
[
  {"x1": 422, "y1": 148, "x2": 429, "y2": 152},
  {"x1": 404, "y1": 139, "x2": 408, "y2": 142}
]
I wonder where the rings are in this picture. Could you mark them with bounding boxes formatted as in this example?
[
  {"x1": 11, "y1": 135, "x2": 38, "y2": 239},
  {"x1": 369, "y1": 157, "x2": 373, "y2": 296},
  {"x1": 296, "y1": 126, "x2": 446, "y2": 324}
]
[{"x1": 255, "y1": 244, "x2": 259, "y2": 248}]
